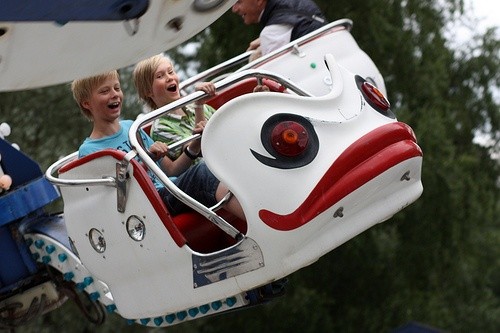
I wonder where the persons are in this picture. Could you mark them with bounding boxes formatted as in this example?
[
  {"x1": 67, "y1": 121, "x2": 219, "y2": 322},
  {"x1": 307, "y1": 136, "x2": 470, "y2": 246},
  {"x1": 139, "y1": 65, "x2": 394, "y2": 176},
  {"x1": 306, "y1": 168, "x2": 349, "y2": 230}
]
[
  {"x1": 71, "y1": 70, "x2": 247, "y2": 221},
  {"x1": 133, "y1": 53, "x2": 269, "y2": 167},
  {"x1": 232, "y1": 0, "x2": 327, "y2": 63}
]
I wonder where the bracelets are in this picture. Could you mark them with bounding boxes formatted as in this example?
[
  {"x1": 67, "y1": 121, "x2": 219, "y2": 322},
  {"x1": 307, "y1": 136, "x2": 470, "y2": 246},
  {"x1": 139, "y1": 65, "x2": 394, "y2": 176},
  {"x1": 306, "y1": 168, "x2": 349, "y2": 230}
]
[{"x1": 184, "y1": 145, "x2": 200, "y2": 160}]
